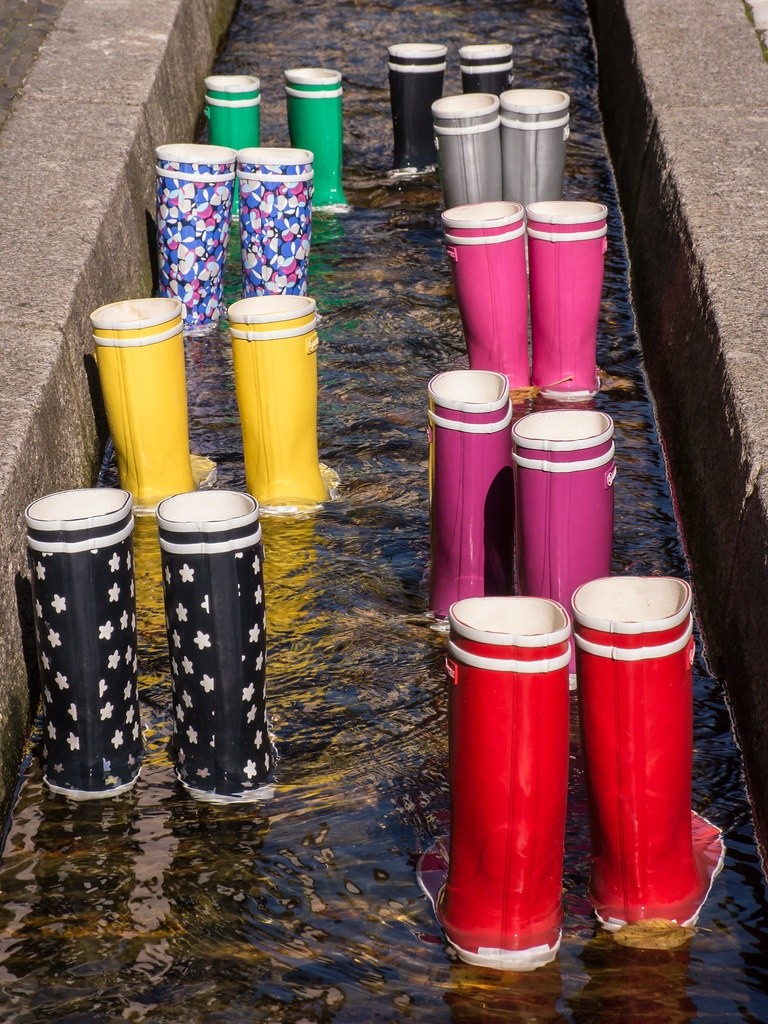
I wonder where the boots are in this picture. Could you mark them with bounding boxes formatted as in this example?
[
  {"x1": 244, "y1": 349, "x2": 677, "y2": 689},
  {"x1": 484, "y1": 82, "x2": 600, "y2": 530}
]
[
  {"x1": 498, "y1": 88, "x2": 570, "y2": 272},
  {"x1": 573, "y1": 573, "x2": 725, "y2": 951},
  {"x1": 24, "y1": 489, "x2": 143, "y2": 802},
  {"x1": 440, "y1": 200, "x2": 530, "y2": 395},
  {"x1": 460, "y1": 43, "x2": 514, "y2": 99},
  {"x1": 228, "y1": 295, "x2": 340, "y2": 518},
  {"x1": 157, "y1": 488, "x2": 279, "y2": 804},
  {"x1": 427, "y1": 370, "x2": 512, "y2": 627},
  {"x1": 285, "y1": 68, "x2": 349, "y2": 215},
  {"x1": 89, "y1": 297, "x2": 219, "y2": 516},
  {"x1": 417, "y1": 594, "x2": 573, "y2": 972},
  {"x1": 156, "y1": 144, "x2": 236, "y2": 338},
  {"x1": 512, "y1": 409, "x2": 616, "y2": 690},
  {"x1": 236, "y1": 146, "x2": 315, "y2": 298},
  {"x1": 203, "y1": 75, "x2": 260, "y2": 220},
  {"x1": 387, "y1": 44, "x2": 446, "y2": 178},
  {"x1": 431, "y1": 92, "x2": 500, "y2": 208},
  {"x1": 526, "y1": 200, "x2": 609, "y2": 404}
]
